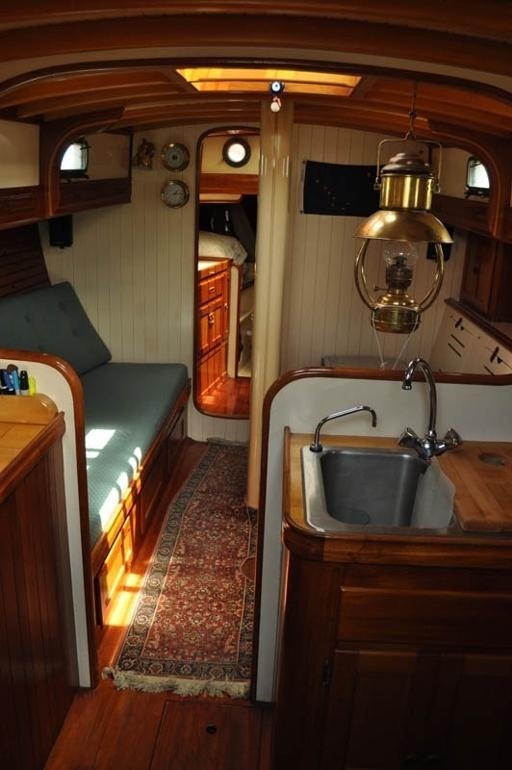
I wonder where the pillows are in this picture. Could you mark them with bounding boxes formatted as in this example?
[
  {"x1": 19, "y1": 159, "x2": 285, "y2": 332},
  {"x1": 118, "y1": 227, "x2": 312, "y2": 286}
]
[{"x1": 198, "y1": 230, "x2": 249, "y2": 266}]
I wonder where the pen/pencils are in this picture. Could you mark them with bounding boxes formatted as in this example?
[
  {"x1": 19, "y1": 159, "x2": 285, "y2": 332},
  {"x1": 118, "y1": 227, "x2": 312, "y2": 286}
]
[{"x1": 3, "y1": 364, "x2": 37, "y2": 396}]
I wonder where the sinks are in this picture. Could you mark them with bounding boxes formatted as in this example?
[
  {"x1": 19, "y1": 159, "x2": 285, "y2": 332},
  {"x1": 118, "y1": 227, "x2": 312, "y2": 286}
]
[{"x1": 302, "y1": 443, "x2": 465, "y2": 537}]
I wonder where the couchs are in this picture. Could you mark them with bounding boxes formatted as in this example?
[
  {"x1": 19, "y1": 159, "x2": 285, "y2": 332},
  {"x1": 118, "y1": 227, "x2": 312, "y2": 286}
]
[{"x1": 0, "y1": 281, "x2": 194, "y2": 626}]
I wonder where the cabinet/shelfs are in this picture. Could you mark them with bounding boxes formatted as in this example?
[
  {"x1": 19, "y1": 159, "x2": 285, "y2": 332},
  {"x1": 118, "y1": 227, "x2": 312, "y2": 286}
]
[
  {"x1": 319, "y1": 586, "x2": 511, "y2": 769},
  {"x1": 428, "y1": 307, "x2": 512, "y2": 375},
  {"x1": 96, "y1": 404, "x2": 189, "y2": 628},
  {"x1": 460, "y1": 232, "x2": 496, "y2": 320},
  {"x1": 196, "y1": 261, "x2": 231, "y2": 401}
]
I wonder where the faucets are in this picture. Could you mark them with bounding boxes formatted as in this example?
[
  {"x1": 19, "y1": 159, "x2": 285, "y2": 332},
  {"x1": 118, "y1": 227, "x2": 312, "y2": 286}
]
[
  {"x1": 401, "y1": 357, "x2": 438, "y2": 434},
  {"x1": 314, "y1": 404, "x2": 377, "y2": 444}
]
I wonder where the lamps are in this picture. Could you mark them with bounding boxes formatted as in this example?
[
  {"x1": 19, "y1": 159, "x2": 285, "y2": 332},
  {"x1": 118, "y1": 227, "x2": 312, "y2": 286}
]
[{"x1": 350, "y1": 79, "x2": 456, "y2": 335}]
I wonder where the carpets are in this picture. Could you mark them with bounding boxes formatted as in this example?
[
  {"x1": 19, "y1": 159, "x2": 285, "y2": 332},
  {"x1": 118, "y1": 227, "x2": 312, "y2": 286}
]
[{"x1": 96, "y1": 435, "x2": 259, "y2": 701}]
